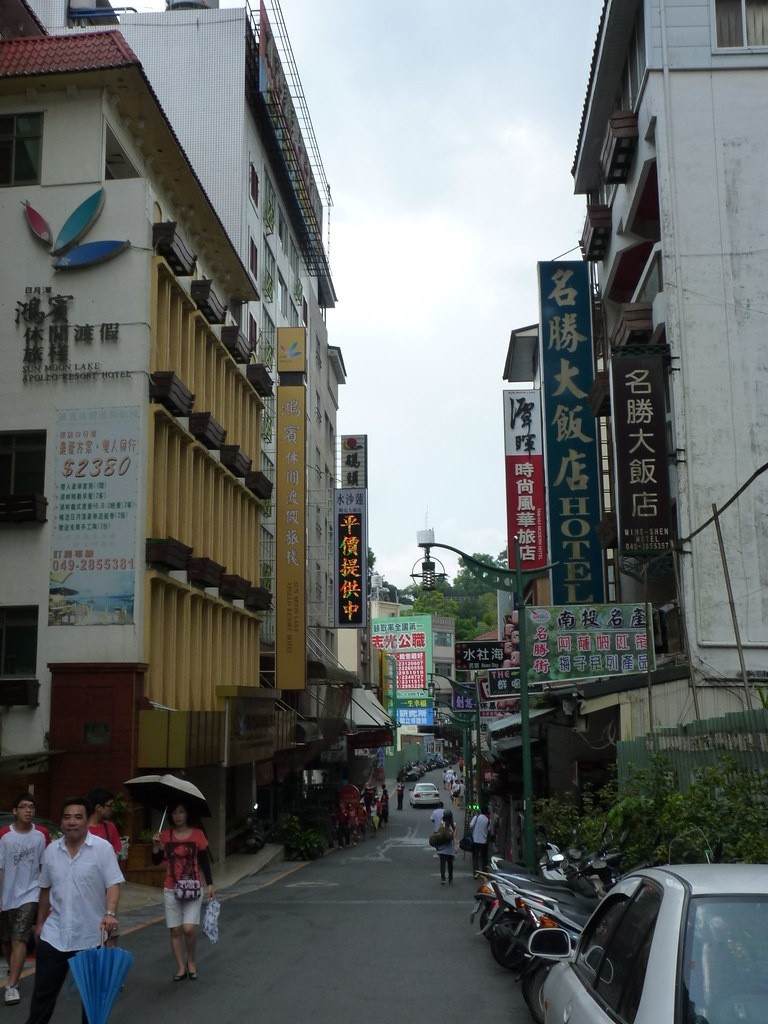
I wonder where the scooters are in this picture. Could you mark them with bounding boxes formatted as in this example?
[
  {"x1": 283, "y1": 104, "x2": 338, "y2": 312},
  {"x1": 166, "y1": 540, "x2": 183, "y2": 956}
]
[
  {"x1": 397, "y1": 751, "x2": 450, "y2": 783},
  {"x1": 469, "y1": 821, "x2": 717, "y2": 1024},
  {"x1": 235, "y1": 803, "x2": 267, "y2": 854}
]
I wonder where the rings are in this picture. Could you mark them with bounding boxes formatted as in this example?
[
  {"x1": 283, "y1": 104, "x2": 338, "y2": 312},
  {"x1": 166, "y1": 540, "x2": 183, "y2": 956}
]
[{"x1": 113, "y1": 928, "x2": 116, "y2": 930}]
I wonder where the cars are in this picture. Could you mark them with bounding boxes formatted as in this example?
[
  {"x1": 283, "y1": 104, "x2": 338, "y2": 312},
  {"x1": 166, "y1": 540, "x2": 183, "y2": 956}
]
[
  {"x1": 527, "y1": 864, "x2": 767, "y2": 1024},
  {"x1": 408, "y1": 782, "x2": 440, "y2": 809}
]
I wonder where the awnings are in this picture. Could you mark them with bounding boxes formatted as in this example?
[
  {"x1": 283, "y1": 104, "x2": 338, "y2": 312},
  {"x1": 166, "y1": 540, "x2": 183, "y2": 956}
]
[
  {"x1": 307, "y1": 628, "x2": 400, "y2": 732},
  {"x1": 485, "y1": 708, "x2": 558, "y2": 737},
  {"x1": 496, "y1": 737, "x2": 539, "y2": 751}
]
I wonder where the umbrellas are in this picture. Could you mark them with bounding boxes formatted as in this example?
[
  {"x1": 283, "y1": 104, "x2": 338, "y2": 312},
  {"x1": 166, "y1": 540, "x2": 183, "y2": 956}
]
[
  {"x1": 67, "y1": 924, "x2": 133, "y2": 1024},
  {"x1": 373, "y1": 816, "x2": 380, "y2": 831},
  {"x1": 124, "y1": 775, "x2": 212, "y2": 843}
]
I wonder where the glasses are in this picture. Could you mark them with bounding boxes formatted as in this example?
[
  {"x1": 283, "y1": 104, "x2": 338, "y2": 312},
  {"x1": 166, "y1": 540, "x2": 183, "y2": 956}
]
[
  {"x1": 104, "y1": 803, "x2": 114, "y2": 807},
  {"x1": 16, "y1": 804, "x2": 35, "y2": 809}
]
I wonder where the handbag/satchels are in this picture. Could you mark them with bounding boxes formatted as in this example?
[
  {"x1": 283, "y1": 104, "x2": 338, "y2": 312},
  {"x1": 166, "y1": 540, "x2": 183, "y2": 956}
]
[
  {"x1": 201, "y1": 898, "x2": 220, "y2": 942},
  {"x1": 117, "y1": 836, "x2": 130, "y2": 859},
  {"x1": 173, "y1": 880, "x2": 201, "y2": 900},
  {"x1": 459, "y1": 834, "x2": 474, "y2": 852},
  {"x1": 430, "y1": 825, "x2": 454, "y2": 850}
]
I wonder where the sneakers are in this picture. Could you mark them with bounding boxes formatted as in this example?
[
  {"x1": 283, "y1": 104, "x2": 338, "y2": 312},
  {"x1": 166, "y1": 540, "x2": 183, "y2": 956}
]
[{"x1": 4, "y1": 984, "x2": 20, "y2": 1005}]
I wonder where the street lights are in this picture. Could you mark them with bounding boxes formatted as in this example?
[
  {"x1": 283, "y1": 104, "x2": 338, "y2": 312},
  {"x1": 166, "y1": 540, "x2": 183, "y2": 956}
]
[
  {"x1": 409, "y1": 542, "x2": 562, "y2": 866},
  {"x1": 422, "y1": 669, "x2": 484, "y2": 838}
]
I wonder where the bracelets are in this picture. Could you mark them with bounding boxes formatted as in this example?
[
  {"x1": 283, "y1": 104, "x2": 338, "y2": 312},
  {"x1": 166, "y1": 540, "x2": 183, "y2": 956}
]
[{"x1": 104, "y1": 911, "x2": 116, "y2": 916}]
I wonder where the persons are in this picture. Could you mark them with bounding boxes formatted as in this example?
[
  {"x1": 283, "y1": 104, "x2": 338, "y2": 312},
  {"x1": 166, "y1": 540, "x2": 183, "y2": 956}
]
[
  {"x1": 88, "y1": 788, "x2": 121, "y2": 860},
  {"x1": 358, "y1": 803, "x2": 367, "y2": 835},
  {"x1": 25, "y1": 797, "x2": 125, "y2": 1024},
  {"x1": 329, "y1": 804, "x2": 351, "y2": 849},
  {"x1": 430, "y1": 803, "x2": 445, "y2": 859},
  {"x1": 443, "y1": 757, "x2": 465, "y2": 803},
  {"x1": 347, "y1": 803, "x2": 359, "y2": 845},
  {"x1": 437, "y1": 810, "x2": 459, "y2": 884},
  {"x1": 469, "y1": 806, "x2": 489, "y2": 879},
  {"x1": 368, "y1": 785, "x2": 389, "y2": 830},
  {"x1": 396, "y1": 781, "x2": 404, "y2": 810},
  {"x1": 151, "y1": 801, "x2": 215, "y2": 981},
  {"x1": 0, "y1": 792, "x2": 51, "y2": 1005}
]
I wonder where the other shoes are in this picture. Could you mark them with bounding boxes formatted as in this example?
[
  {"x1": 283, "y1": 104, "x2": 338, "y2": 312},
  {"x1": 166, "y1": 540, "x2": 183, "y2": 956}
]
[
  {"x1": 474, "y1": 872, "x2": 479, "y2": 878},
  {"x1": 440, "y1": 880, "x2": 446, "y2": 884},
  {"x1": 339, "y1": 845, "x2": 342, "y2": 848},
  {"x1": 173, "y1": 969, "x2": 186, "y2": 980},
  {"x1": 353, "y1": 843, "x2": 356, "y2": 845},
  {"x1": 187, "y1": 962, "x2": 198, "y2": 978},
  {"x1": 108, "y1": 929, "x2": 119, "y2": 936}
]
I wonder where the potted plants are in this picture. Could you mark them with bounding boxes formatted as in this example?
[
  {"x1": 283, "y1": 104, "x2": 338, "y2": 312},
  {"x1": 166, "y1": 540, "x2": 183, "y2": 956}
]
[{"x1": 127, "y1": 827, "x2": 160, "y2": 869}]
